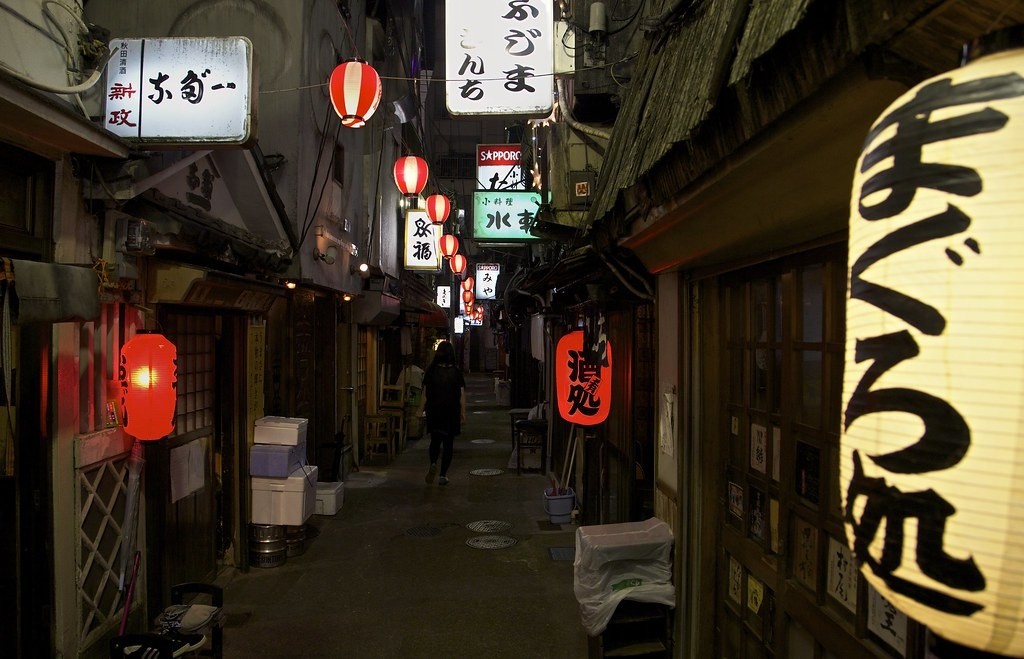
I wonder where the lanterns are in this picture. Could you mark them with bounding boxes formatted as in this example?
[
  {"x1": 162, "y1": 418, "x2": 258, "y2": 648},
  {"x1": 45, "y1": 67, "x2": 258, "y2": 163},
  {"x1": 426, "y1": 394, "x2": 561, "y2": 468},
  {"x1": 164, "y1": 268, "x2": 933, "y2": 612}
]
[
  {"x1": 116, "y1": 328, "x2": 178, "y2": 442},
  {"x1": 425, "y1": 193, "x2": 451, "y2": 226},
  {"x1": 554, "y1": 316, "x2": 613, "y2": 426},
  {"x1": 439, "y1": 232, "x2": 459, "y2": 260},
  {"x1": 449, "y1": 253, "x2": 467, "y2": 275},
  {"x1": 392, "y1": 155, "x2": 430, "y2": 199},
  {"x1": 461, "y1": 277, "x2": 484, "y2": 321},
  {"x1": 836, "y1": 44, "x2": 1024, "y2": 659},
  {"x1": 328, "y1": 58, "x2": 383, "y2": 128}
]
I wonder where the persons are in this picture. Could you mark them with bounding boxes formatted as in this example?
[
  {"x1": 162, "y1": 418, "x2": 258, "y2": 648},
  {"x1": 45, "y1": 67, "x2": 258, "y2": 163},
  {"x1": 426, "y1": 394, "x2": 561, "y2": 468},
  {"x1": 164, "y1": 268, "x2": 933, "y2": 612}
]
[{"x1": 415, "y1": 341, "x2": 466, "y2": 488}]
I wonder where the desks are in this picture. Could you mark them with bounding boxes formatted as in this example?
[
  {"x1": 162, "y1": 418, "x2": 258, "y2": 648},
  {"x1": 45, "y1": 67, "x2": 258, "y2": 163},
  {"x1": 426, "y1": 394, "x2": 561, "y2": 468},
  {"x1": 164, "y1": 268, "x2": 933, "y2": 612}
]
[{"x1": 574, "y1": 516, "x2": 676, "y2": 659}]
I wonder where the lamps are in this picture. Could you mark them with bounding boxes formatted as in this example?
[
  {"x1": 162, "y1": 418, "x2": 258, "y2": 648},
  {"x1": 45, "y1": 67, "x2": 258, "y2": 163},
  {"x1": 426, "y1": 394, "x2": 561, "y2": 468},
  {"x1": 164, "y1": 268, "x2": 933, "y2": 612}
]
[
  {"x1": 554, "y1": 319, "x2": 613, "y2": 426},
  {"x1": 839, "y1": 23, "x2": 1024, "y2": 659},
  {"x1": 454, "y1": 315, "x2": 463, "y2": 339},
  {"x1": 449, "y1": 250, "x2": 467, "y2": 275},
  {"x1": 438, "y1": 229, "x2": 459, "y2": 259},
  {"x1": 118, "y1": 329, "x2": 179, "y2": 445},
  {"x1": 328, "y1": 57, "x2": 383, "y2": 129},
  {"x1": 425, "y1": 194, "x2": 451, "y2": 225},
  {"x1": 393, "y1": 152, "x2": 429, "y2": 199},
  {"x1": 460, "y1": 276, "x2": 484, "y2": 322}
]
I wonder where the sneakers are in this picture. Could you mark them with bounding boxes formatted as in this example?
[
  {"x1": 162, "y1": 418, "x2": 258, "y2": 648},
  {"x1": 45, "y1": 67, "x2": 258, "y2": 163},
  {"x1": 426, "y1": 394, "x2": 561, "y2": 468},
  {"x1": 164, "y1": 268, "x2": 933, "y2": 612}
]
[
  {"x1": 439, "y1": 477, "x2": 450, "y2": 485},
  {"x1": 425, "y1": 464, "x2": 436, "y2": 484}
]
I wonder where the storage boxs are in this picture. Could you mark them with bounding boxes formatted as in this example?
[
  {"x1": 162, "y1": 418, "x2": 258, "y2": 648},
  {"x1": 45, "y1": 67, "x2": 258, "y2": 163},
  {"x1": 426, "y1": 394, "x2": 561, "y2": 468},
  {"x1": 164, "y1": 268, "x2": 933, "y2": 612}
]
[
  {"x1": 249, "y1": 415, "x2": 346, "y2": 569},
  {"x1": 542, "y1": 487, "x2": 577, "y2": 524}
]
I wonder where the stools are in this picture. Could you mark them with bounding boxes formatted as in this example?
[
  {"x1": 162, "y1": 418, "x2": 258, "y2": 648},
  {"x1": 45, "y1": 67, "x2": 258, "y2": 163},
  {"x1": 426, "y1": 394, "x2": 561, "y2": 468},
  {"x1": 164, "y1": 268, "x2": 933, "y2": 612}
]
[
  {"x1": 363, "y1": 362, "x2": 411, "y2": 466},
  {"x1": 509, "y1": 408, "x2": 537, "y2": 455},
  {"x1": 515, "y1": 419, "x2": 549, "y2": 476}
]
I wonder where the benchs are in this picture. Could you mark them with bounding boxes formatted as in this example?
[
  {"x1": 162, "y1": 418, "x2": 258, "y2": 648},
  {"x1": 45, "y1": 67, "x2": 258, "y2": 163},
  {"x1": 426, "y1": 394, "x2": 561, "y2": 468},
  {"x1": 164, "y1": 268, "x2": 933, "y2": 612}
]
[{"x1": 108, "y1": 582, "x2": 223, "y2": 659}]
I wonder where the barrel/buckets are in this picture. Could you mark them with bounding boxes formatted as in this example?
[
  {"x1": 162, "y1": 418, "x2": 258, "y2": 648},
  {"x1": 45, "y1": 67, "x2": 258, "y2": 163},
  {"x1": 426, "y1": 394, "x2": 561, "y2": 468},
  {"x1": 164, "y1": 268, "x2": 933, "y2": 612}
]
[
  {"x1": 252, "y1": 522, "x2": 285, "y2": 570},
  {"x1": 283, "y1": 524, "x2": 307, "y2": 557}
]
